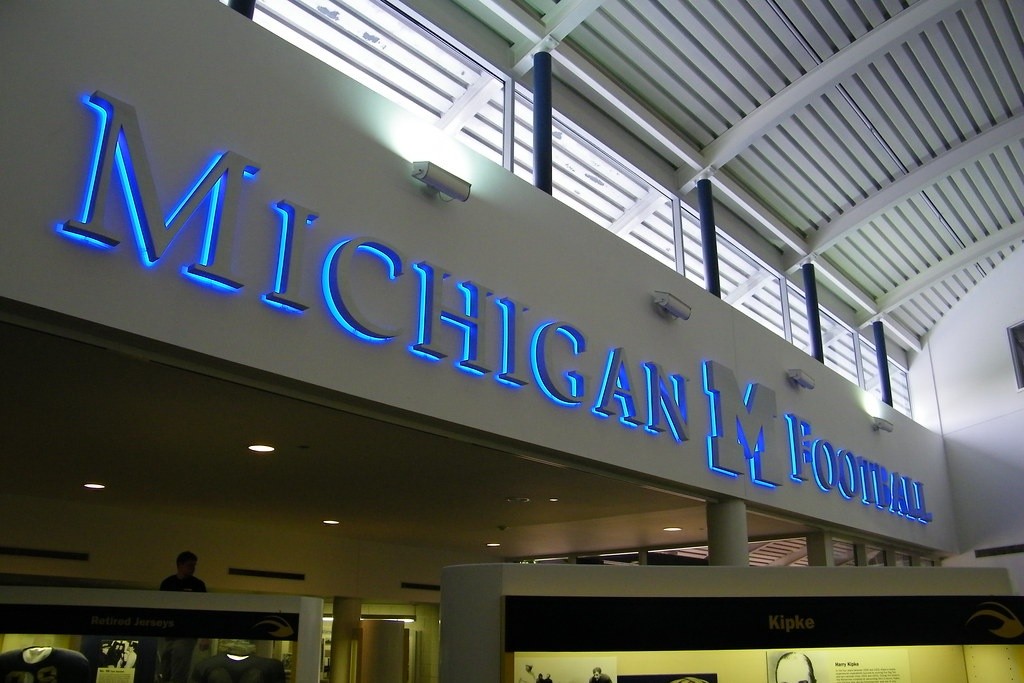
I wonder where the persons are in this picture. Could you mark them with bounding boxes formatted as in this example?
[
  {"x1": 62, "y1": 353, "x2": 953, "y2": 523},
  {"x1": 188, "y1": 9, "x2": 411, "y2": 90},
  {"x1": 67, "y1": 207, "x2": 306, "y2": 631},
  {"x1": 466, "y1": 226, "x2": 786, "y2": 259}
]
[
  {"x1": 776, "y1": 652, "x2": 817, "y2": 683},
  {"x1": 536, "y1": 673, "x2": 552, "y2": 683},
  {"x1": 589, "y1": 667, "x2": 612, "y2": 683},
  {"x1": 159, "y1": 551, "x2": 212, "y2": 683},
  {"x1": 98, "y1": 642, "x2": 136, "y2": 669}
]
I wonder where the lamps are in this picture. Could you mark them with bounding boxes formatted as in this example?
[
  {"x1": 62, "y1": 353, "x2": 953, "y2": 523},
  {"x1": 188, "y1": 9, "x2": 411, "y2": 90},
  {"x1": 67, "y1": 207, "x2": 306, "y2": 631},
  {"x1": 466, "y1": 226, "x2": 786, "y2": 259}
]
[
  {"x1": 653, "y1": 290, "x2": 692, "y2": 321},
  {"x1": 410, "y1": 160, "x2": 472, "y2": 203},
  {"x1": 788, "y1": 368, "x2": 816, "y2": 390},
  {"x1": 872, "y1": 416, "x2": 894, "y2": 433}
]
